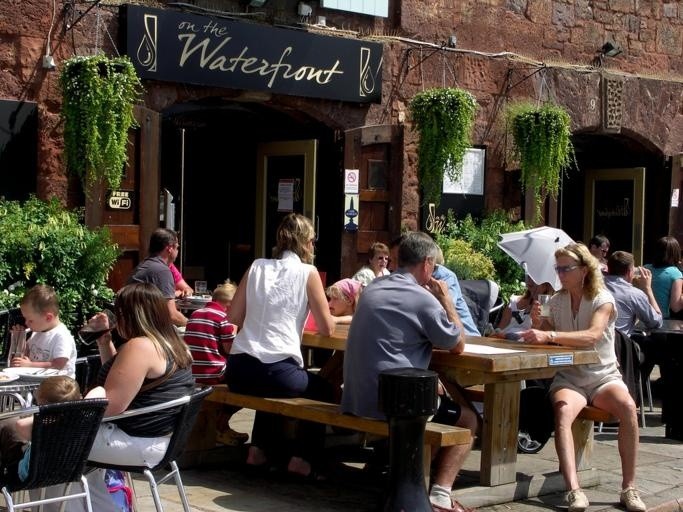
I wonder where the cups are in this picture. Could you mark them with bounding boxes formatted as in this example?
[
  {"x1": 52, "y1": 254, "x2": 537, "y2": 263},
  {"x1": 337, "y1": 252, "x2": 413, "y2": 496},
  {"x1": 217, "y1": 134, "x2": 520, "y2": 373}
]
[
  {"x1": 195, "y1": 281, "x2": 207, "y2": 296},
  {"x1": 78, "y1": 309, "x2": 116, "y2": 345},
  {"x1": 538, "y1": 294, "x2": 551, "y2": 317},
  {"x1": 634, "y1": 267, "x2": 641, "y2": 278},
  {"x1": 10, "y1": 330, "x2": 26, "y2": 359}
]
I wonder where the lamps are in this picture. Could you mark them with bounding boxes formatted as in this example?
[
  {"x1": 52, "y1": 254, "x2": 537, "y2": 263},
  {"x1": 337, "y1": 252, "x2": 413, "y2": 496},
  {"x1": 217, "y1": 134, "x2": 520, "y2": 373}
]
[
  {"x1": 592, "y1": 41, "x2": 622, "y2": 67},
  {"x1": 409, "y1": 36, "x2": 457, "y2": 72}
]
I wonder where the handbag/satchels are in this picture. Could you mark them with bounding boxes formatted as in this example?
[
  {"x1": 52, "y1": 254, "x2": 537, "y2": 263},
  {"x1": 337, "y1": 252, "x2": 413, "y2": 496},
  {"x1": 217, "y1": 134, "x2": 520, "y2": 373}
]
[
  {"x1": 515, "y1": 385, "x2": 555, "y2": 455},
  {"x1": 103, "y1": 467, "x2": 134, "y2": 512}
]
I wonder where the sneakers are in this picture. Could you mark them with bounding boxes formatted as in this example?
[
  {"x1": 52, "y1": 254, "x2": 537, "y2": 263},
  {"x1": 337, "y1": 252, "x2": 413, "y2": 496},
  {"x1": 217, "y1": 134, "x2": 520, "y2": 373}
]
[
  {"x1": 564, "y1": 487, "x2": 590, "y2": 512},
  {"x1": 620, "y1": 486, "x2": 646, "y2": 512}
]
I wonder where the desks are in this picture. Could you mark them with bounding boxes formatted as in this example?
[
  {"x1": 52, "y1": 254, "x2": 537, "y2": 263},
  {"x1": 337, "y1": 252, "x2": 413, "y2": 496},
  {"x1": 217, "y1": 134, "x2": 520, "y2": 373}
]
[
  {"x1": 303, "y1": 318, "x2": 599, "y2": 508},
  {"x1": 633, "y1": 319, "x2": 683, "y2": 439}
]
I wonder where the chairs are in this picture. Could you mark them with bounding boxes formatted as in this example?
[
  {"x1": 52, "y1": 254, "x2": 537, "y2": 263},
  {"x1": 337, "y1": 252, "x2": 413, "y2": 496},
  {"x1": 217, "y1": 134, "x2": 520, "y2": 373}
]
[{"x1": 599, "y1": 328, "x2": 654, "y2": 434}]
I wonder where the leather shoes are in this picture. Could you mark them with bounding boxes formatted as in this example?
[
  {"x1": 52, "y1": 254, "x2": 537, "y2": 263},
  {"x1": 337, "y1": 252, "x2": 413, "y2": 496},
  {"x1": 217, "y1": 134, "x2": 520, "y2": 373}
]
[{"x1": 430, "y1": 498, "x2": 476, "y2": 512}]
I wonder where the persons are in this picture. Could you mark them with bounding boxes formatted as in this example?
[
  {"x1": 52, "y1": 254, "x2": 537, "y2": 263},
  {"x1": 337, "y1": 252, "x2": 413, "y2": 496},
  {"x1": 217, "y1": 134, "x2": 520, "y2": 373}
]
[
  {"x1": 8, "y1": 286, "x2": 77, "y2": 401},
  {"x1": 184, "y1": 283, "x2": 249, "y2": 446},
  {"x1": 521, "y1": 243, "x2": 647, "y2": 510},
  {"x1": 328, "y1": 228, "x2": 682, "y2": 445},
  {"x1": 167, "y1": 259, "x2": 193, "y2": 299},
  {"x1": 337, "y1": 231, "x2": 479, "y2": 512},
  {"x1": 29, "y1": 283, "x2": 195, "y2": 512},
  {"x1": 124, "y1": 226, "x2": 189, "y2": 338},
  {"x1": 0, "y1": 375, "x2": 83, "y2": 486},
  {"x1": 227, "y1": 214, "x2": 335, "y2": 489}
]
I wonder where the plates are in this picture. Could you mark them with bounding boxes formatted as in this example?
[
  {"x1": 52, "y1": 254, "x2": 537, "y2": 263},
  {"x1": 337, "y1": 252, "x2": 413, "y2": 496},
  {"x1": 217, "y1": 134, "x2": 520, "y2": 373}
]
[
  {"x1": 0, "y1": 367, "x2": 67, "y2": 383},
  {"x1": 186, "y1": 295, "x2": 212, "y2": 304}
]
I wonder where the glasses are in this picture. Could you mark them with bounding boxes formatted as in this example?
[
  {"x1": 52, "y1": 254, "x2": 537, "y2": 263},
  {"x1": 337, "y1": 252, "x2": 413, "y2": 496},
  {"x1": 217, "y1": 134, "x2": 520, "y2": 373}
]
[
  {"x1": 377, "y1": 256, "x2": 388, "y2": 260},
  {"x1": 555, "y1": 264, "x2": 584, "y2": 272}
]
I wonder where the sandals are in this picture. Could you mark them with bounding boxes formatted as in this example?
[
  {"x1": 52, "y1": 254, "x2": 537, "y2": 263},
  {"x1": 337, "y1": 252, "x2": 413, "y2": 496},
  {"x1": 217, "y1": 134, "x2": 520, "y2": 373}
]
[
  {"x1": 215, "y1": 430, "x2": 239, "y2": 447},
  {"x1": 226, "y1": 427, "x2": 249, "y2": 443},
  {"x1": 245, "y1": 457, "x2": 286, "y2": 481},
  {"x1": 286, "y1": 464, "x2": 331, "y2": 490}
]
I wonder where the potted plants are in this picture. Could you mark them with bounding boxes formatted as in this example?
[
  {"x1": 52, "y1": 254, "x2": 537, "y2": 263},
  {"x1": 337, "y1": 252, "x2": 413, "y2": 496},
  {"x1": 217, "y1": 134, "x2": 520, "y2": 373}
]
[
  {"x1": 409, "y1": 85, "x2": 480, "y2": 209},
  {"x1": 503, "y1": 97, "x2": 580, "y2": 226},
  {"x1": 55, "y1": 50, "x2": 148, "y2": 209}
]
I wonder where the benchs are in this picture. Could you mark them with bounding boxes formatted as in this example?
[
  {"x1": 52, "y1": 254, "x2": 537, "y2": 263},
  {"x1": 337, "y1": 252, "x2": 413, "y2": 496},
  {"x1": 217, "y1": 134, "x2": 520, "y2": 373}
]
[
  {"x1": 203, "y1": 382, "x2": 472, "y2": 511},
  {"x1": 466, "y1": 384, "x2": 620, "y2": 491}
]
